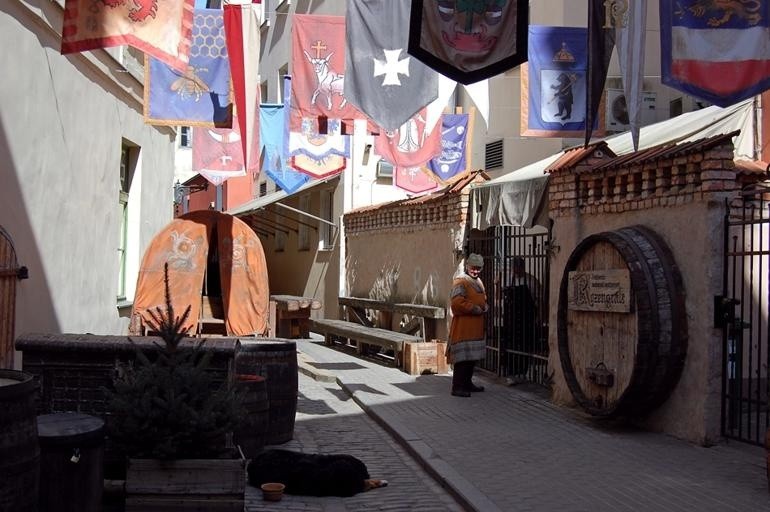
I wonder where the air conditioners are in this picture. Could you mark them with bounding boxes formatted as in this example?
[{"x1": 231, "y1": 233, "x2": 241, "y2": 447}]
[{"x1": 596, "y1": 89, "x2": 659, "y2": 135}]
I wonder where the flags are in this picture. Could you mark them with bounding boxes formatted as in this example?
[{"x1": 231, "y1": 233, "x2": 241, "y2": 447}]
[
  {"x1": 190, "y1": 73, "x2": 263, "y2": 178},
  {"x1": 519, "y1": 22, "x2": 607, "y2": 140},
  {"x1": 288, "y1": 13, "x2": 371, "y2": 123},
  {"x1": 141, "y1": 5, "x2": 234, "y2": 130}
]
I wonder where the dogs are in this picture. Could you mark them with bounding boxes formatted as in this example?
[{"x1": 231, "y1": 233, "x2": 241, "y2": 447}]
[{"x1": 247, "y1": 447, "x2": 389, "y2": 498}]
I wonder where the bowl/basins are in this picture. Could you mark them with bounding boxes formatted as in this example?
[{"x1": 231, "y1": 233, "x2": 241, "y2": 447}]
[{"x1": 261, "y1": 482, "x2": 286, "y2": 502}]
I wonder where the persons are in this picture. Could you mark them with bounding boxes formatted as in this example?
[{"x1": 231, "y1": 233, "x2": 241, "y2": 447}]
[
  {"x1": 443, "y1": 251, "x2": 490, "y2": 396},
  {"x1": 499, "y1": 254, "x2": 544, "y2": 388}
]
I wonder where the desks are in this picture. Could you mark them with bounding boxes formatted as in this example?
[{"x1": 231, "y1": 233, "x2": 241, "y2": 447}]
[{"x1": 35, "y1": 411, "x2": 105, "y2": 511}]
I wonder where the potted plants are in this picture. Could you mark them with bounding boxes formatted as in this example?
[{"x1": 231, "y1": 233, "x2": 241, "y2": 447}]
[{"x1": 94, "y1": 259, "x2": 250, "y2": 464}]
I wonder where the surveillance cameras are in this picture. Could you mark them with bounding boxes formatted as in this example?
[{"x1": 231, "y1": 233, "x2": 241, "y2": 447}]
[{"x1": 365, "y1": 142, "x2": 372, "y2": 149}]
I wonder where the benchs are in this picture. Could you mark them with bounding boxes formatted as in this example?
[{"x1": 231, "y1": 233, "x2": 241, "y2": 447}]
[{"x1": 307, "y1": 296, "x2": 446, "y2": 368}]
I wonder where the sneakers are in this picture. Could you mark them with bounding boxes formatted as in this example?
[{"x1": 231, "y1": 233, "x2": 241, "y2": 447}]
[
  {"x1": 451, "y1": 383, "x2": 485, "y2": 397},
  {"x1": 507, "y1": 374, "x2": 524, "y2": 386}
]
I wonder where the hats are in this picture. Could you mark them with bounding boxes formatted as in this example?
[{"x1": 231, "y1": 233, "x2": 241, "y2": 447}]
[{"x1": 467, "y1": 253, "x2": 485, "y2": 268}]
